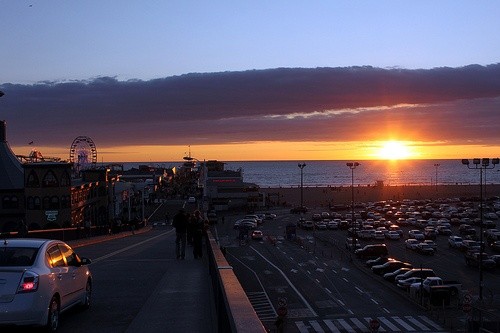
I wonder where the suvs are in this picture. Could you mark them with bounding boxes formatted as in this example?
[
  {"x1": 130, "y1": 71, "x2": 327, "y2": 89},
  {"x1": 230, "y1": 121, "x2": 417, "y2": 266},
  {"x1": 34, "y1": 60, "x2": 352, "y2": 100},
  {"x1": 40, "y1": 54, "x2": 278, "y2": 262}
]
[
  {"x1": 394, "y1": 268, "x2": 437, "y2": 285},
  {"x1": 354, "y1": 243, "x2": 389, "y2": 259}
]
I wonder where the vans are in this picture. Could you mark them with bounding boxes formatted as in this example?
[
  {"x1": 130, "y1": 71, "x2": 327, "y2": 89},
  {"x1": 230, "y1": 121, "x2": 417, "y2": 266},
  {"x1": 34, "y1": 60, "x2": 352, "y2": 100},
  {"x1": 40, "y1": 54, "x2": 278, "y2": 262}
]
[{"x1": 207, "y1": 209, "x2": 218, "y2": 226}]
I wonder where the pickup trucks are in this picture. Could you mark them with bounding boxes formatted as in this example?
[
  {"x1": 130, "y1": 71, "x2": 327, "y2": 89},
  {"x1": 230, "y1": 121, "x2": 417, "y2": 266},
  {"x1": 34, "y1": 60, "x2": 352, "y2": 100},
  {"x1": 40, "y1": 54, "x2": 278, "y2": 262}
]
[{"x1": 409, "y1": 276, "x2": 463, "y2": 299}]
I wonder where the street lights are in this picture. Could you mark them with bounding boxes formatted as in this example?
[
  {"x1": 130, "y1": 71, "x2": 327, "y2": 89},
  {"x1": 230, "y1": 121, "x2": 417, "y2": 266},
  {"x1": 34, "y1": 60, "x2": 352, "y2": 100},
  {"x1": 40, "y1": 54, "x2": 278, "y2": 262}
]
[
  {"x1": 346, "y1": 162, "x2": 359, "y2": 248},
  {"x1": 433, "y1": 163, "x2": 440, "y2": 201},
  {"x1": 297, "y1": 163, "x2": 307, "y2": 230},
  {"x1": 462, "y1": 157, "x2": 500, "y2": 300}
]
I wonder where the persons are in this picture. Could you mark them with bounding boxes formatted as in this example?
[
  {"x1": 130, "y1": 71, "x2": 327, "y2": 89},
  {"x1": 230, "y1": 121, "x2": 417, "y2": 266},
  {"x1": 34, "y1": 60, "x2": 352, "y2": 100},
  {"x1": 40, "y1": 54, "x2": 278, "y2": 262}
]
[
  {"x1": 173, "y1": 208, "x2": 205, "y2": 260},
  {"x1": 222, "y1": 216, "x2": 225, "y2": 223}
]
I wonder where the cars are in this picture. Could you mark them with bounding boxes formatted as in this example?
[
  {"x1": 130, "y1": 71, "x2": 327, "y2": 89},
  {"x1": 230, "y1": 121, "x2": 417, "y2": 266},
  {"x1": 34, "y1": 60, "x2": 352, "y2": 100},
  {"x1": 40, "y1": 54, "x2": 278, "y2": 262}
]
[
  {"x1": 365, "y1": 256, "x2": 395, "y2": 269},
  {"x1": 0, "y1": 236, "x2": 93, "y2": 333},
  {"x1": 383, "y1": 268, "x2": 411, "y2": 283},
  {"x1": 345, "y1": 237, "x2": 361, "y2": 253},
  {"x1": 251, "y1": 230, "x2": 264, "y2": 240},
  {"x1": 233, "y1": 213, "x2": 276, "y2": 229},
  {"x1": 291, "y1": 195, "x2": 500, "y2": 274},
  {"x1": 397, "y1": 278, "x2": 424, "y2": 293},
  {"x1": 371, "y1": 260, "x2": 412, "y2": 277},
  {"x1": 188, "y1": 196, "x2": 196, "y2": 203}
]
